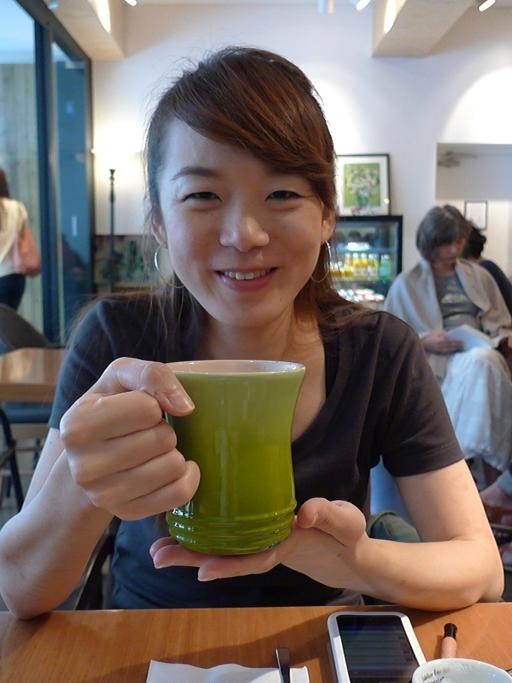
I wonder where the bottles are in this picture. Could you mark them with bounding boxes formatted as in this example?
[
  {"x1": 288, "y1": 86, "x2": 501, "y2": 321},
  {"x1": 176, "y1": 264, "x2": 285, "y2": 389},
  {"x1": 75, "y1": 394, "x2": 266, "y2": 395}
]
[{"x1": 329, "y1": 252, "x2": 393, "y2": 281}]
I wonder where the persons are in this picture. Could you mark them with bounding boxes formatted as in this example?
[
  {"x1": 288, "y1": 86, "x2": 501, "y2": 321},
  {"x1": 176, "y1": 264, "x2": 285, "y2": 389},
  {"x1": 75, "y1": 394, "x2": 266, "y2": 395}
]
[
  {"x1": 1, "y1": 44, "x2": 506, "y2": 621},
  {"x1": 1, "y1": 170, "x2": 30, "y2": 312},
  {"x1": 382, "y1": 203, "x2": 511, "y2": 528}
]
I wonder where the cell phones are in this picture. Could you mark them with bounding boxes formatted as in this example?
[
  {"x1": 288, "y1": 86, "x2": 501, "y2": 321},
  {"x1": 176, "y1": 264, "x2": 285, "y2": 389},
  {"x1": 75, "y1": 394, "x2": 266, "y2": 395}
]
[{"x1": 326, "y1": 611, "x2": 429, "y2": 683}]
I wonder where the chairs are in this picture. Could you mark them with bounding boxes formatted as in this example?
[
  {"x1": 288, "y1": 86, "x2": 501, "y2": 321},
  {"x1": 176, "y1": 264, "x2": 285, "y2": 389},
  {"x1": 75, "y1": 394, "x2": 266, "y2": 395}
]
[{"x1": 0, "y1": 303, "x2": 62, "y2": 511}]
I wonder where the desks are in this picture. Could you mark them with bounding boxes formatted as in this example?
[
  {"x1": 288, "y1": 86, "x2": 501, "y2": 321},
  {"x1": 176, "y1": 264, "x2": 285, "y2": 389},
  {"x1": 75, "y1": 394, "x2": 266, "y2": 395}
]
[
  {"x1": 0, "y1": 346, "x2": 71, "y2": 403},
  {"x1": 0, "y1": 602, "x2": 512, "y2": 683}
]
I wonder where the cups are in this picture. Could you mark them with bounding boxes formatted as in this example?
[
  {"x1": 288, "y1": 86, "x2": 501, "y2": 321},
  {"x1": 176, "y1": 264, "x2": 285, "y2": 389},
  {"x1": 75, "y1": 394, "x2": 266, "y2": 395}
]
[{"x1": 161, "y1": 359, "x2": 308, "y2": 558}]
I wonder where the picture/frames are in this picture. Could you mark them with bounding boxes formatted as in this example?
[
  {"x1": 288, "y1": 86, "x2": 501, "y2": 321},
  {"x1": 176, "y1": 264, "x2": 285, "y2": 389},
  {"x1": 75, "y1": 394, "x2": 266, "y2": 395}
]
[
  {"x1": 465, "y1": 200, "x2": 489, "y2": 231},
  {"x1": 334, "y1": 153, "x2": 391, "y2": 216}
]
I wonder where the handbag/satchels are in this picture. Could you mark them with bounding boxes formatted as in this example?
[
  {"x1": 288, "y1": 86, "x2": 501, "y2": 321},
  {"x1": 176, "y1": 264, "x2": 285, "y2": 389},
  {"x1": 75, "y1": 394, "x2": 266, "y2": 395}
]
[{"x1": 12, "y1": 225, "x2": 40, "y2": 277}]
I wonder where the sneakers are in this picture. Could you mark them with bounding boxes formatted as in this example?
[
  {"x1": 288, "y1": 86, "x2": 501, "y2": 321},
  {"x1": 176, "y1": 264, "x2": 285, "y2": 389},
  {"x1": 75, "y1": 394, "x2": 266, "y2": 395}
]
[{"x1": 482, "y1": 498, "x2": 511, "y2": 569}]
